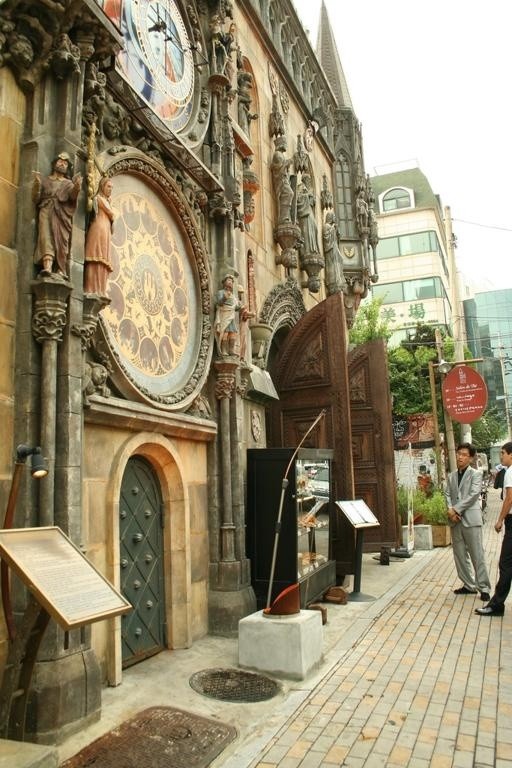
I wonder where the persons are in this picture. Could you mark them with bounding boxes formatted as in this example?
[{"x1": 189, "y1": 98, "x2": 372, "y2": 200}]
[
  {"x1": 212, "y1": 272, "x2": 248, "y2": 357},
  {"x1": 321, "y1": 212, "x2": 348, "y2": 290},
  {"x1": 83, "y1": 176, "x2": 118, "y2": 300},
  {"x1": 443, "y1": 443, "x2": 491, "y2": 601},
  {"x1": 32, "y1": 150, "x2": 82, "y2": 280},
  {"x1": 237, "y1": 72, "x2": 259, "y2": 139},
  {"x1": 236, "y1": 284, "x2": 256, "y2": 362},
  {"x1": 271, "y1": 136, "x2": 295, "y2": 225},
  {"x1": 474, "y1": 441, "x2": 511, "y2": 616},
  {"x1": 495, "y1": 463, "x2": 508, "y2": 500},
  {"x1": 354, "y1": 190, "x2": 369, "y2": 228},
  {"x1": 296, "y1": 172, "x2": 320, "y2": 255}
]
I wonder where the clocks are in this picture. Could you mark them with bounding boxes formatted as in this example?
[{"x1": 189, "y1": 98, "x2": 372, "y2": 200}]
[{"x1": 91, "y1": 0, "x2": 212, "y2": 154}]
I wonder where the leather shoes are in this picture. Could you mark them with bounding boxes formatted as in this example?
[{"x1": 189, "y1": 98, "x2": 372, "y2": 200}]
[
  {"x1": 481, "y1": 592, "x2": 489, "y2": 601},
  {"x1": 454, "y1": 587, "x2": 477, "y2": 594},
  {"x1": 475, "y1": 604, "x2": 504, "y2": 616}
]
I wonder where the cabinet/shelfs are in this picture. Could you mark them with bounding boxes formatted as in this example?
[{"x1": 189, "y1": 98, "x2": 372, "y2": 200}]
[{"x1": 245, "y1": 448, "x2": 337, "y2": 609}]
